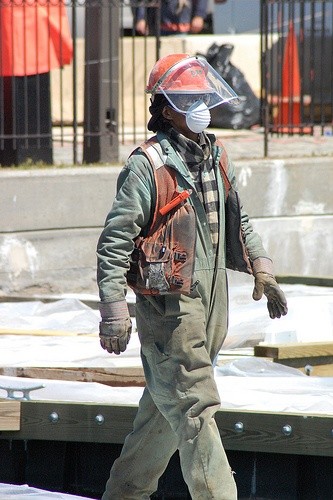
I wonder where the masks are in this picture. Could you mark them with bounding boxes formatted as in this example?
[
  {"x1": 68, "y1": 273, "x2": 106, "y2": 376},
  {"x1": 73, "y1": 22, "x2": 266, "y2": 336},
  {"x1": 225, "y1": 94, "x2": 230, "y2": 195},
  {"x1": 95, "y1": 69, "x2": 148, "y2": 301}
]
[{"x1": 166, "y1": 100, "x2": 212, "y2": 134}]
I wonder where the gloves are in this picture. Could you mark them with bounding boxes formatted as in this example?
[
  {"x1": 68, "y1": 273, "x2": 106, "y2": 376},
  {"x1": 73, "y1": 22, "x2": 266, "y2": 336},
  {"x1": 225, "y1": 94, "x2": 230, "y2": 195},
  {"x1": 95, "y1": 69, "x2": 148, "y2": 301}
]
[
  {"x1": 95, "y1": 300, "x2": 133, "y2": 354},
  {"x1": 248, "y1": 259, "x2": 291, "y2": 319}
]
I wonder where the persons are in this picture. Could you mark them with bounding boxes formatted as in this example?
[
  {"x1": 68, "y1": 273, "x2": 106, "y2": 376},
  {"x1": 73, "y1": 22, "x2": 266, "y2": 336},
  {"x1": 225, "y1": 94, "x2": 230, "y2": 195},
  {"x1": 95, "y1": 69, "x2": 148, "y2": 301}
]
[
  {"x1": 96, "y1": 54, "x2": 287, "y2": 500},
  {"x1": 134, "y1": 1, "x2": 209, "y2": 35}
]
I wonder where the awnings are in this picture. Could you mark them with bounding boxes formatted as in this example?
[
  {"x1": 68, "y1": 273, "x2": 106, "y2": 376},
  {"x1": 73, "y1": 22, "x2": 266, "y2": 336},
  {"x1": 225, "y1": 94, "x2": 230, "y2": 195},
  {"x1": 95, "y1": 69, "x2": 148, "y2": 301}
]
[{"x1": 1, "y1": 0, "x2": 71, "y2": 76}]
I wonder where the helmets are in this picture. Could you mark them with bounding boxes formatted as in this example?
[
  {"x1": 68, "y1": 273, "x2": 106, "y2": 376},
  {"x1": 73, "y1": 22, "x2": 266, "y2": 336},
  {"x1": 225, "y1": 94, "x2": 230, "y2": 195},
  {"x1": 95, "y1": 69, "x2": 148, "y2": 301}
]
[{"x1": 145, "y1": 52, "x2": 240, "y2": 114}]
